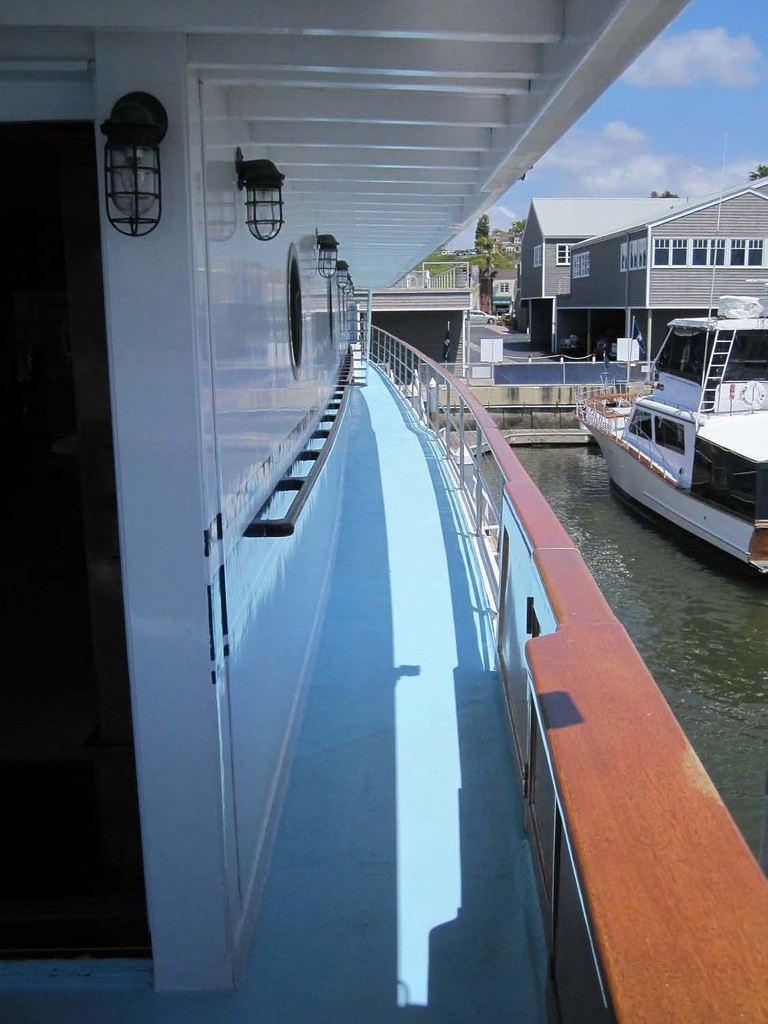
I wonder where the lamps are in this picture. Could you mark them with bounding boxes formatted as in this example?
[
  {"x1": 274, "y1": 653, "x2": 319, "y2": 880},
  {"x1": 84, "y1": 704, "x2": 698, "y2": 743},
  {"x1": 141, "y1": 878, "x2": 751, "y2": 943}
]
[
  {"x1": 235, "y1": 147, "x2": 285, "y2": 242},
  {"x1": 316, "y1": 234, "x2": 356, "y2": 298},
  {"x1": 99, "y1": 89, "x2": 170, "y2": 239}
]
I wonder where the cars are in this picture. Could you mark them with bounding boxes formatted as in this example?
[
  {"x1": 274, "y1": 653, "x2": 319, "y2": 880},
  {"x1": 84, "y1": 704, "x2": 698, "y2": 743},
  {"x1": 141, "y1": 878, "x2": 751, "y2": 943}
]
[
  {"x1": 465, "y1": 310, "x2": 497, "y2": 325},
  {"x1": 455, "y1": 249, "x2": 477, "y2": 256}
]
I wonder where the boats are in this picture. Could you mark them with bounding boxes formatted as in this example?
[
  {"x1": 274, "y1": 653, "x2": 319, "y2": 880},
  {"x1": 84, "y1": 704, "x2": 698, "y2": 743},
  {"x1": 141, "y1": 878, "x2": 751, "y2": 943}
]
[{"x1": 574, "y1": 315, "x2": 768, "y2": 575}]
